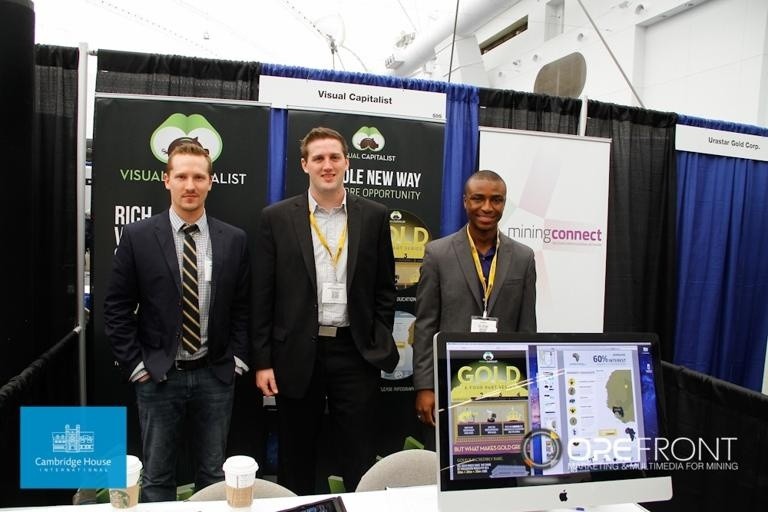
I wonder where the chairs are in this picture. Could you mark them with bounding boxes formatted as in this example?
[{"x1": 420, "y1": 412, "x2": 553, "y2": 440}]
[{"x1": 189, "y1": 448, "x2": 440, "y2": 505}]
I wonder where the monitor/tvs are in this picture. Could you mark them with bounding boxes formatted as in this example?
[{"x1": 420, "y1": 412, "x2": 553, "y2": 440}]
[{"x1": 433, "y1": 331, "x2": 673, "y2": 512}]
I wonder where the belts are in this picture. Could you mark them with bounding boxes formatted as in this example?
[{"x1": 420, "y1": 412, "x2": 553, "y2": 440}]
[
  {"x1": 171, "y1": 355, "x2": 207, "y2": 373},
  {"x1": 316, "y1": 324, "x2": 352, "y2": 340}
]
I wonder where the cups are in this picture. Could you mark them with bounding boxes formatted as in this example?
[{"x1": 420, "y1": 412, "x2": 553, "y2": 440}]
[
  {"x1": 108, "y1": 455, "x2": 143, "y2": 509},
  {"x1": 222, "y1": 454, "x2": 260, "y2": 508}
]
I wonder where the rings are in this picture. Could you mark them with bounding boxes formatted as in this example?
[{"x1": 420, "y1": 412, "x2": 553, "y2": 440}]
[{"x1": 416, "y1": 415, "x2": 421, "y2": 418}]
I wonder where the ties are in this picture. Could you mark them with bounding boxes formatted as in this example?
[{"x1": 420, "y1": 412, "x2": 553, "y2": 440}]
[{"x1": 180, "y1": 223, "x2": 202, "y2": 356}]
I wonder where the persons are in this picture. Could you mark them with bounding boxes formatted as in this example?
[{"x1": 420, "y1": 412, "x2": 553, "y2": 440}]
[
  {"x1": 99, "y1": 142, "x2": 252, "y2": 504},
  {"x1": 410, "y1": 169, "x2": 539, "y2": 452},
  {"x1": 244, "y1": 125, "x2": 402, "y2": 499}
]
